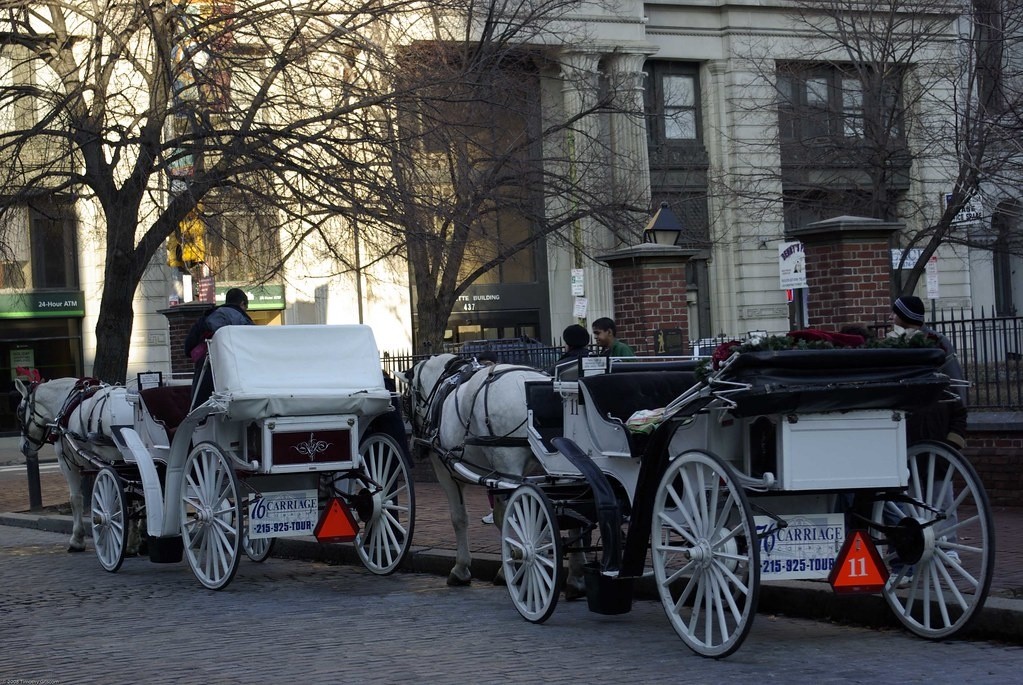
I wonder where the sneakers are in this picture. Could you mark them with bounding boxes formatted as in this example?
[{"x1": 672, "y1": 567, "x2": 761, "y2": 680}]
[
  {"x1": 943, "y1": 551, "x2": 961, "y2": 568},
  {"x1": 888, "y1": 573, "x2": 914, "y2": 585}
]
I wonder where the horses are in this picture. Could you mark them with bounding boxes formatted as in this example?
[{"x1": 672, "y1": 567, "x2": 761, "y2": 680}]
[
  {"x1": 15, "y1": 376, "x2": 147, "y2": 557},
  {"x1": 389, "y1": 354, "x2": 592, "y2": 602}
]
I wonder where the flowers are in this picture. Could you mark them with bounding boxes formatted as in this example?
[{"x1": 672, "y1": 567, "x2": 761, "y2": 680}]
[{"x1": 695, "y1": 324, "x2": 948, "y2": 379}]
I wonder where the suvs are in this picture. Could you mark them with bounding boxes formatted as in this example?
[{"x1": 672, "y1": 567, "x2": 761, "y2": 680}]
[{"x1": 450, "y1": 334, "x2": 563, "y2": 372}]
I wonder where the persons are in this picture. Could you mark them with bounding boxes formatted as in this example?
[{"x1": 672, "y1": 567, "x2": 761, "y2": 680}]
[
  {"x1": 888, "y1": 295, "x2": 969, "y2": 451},
  {"x1": 592, "y1": 317, "x2": 640, "y2": 362},
  {"x1": 184, "y1": 288, "x2": 256, "y2": 413},
  {"x1": 547, "y1": 324, "x2": 595, "y2": 377},
  {"x1": 478, "y1": 351, "x2": 500, "y2": 525}
]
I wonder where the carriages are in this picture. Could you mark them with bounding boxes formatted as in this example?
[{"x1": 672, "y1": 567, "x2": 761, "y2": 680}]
[
  {"x1": 387, "y1": 329, "x2": 997, "y2": 661},
  {"x1": 12, "y1": 323, "x2": 418, "y2": 592}
]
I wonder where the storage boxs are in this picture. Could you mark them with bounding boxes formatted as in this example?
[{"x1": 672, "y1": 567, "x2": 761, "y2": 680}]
[
  {"x1": 743, "y1": 409, "x2": 909, "y2": 491},
  {"x1": 242, "y1": 413, "x2": 360, "y2": 474}
]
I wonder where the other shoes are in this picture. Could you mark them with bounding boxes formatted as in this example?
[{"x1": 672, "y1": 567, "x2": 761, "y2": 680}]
[
  {"x1": 482, "y1": 512, "x2": 495, "y2": 524},
  {"x1": 512, "y1": 514, "x2": 518, "y2": 522},
  {"x1": 563, "y1": 536, "x2": 592, "y2": 552}
]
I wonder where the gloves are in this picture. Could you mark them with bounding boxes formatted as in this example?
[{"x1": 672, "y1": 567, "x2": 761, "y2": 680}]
[{"x1": 946, "y1": 440, "x2": 961, "y2": 450}]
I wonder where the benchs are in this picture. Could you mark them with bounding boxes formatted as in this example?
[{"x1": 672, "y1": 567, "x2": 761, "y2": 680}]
[
  {"x1": 579, "y1": 372, "x2": 702, "y2": 457},
  {"x1": 211, "y1": 324, "x2": 385, "y2": 392},
  {"x1": 525, "y1": 381, "x2": 565, "y2": 453},
  {"x1": 138, "y1": 386, "x2": 196, "y2": 449}
]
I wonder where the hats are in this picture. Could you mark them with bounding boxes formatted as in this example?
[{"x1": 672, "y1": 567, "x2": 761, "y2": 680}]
[
  {"x1": 477, "y1": 351, "x2": 497, "y2": 364},
  {"x1": 893, "y1": 296, "x2": 925, "y2": 326},
  {"x1": 226, "y1": 288, "x2": 247, "y2": 306},
  {"x1": 563, "y1": 324, "x2": 590, "y2": 348}
]
